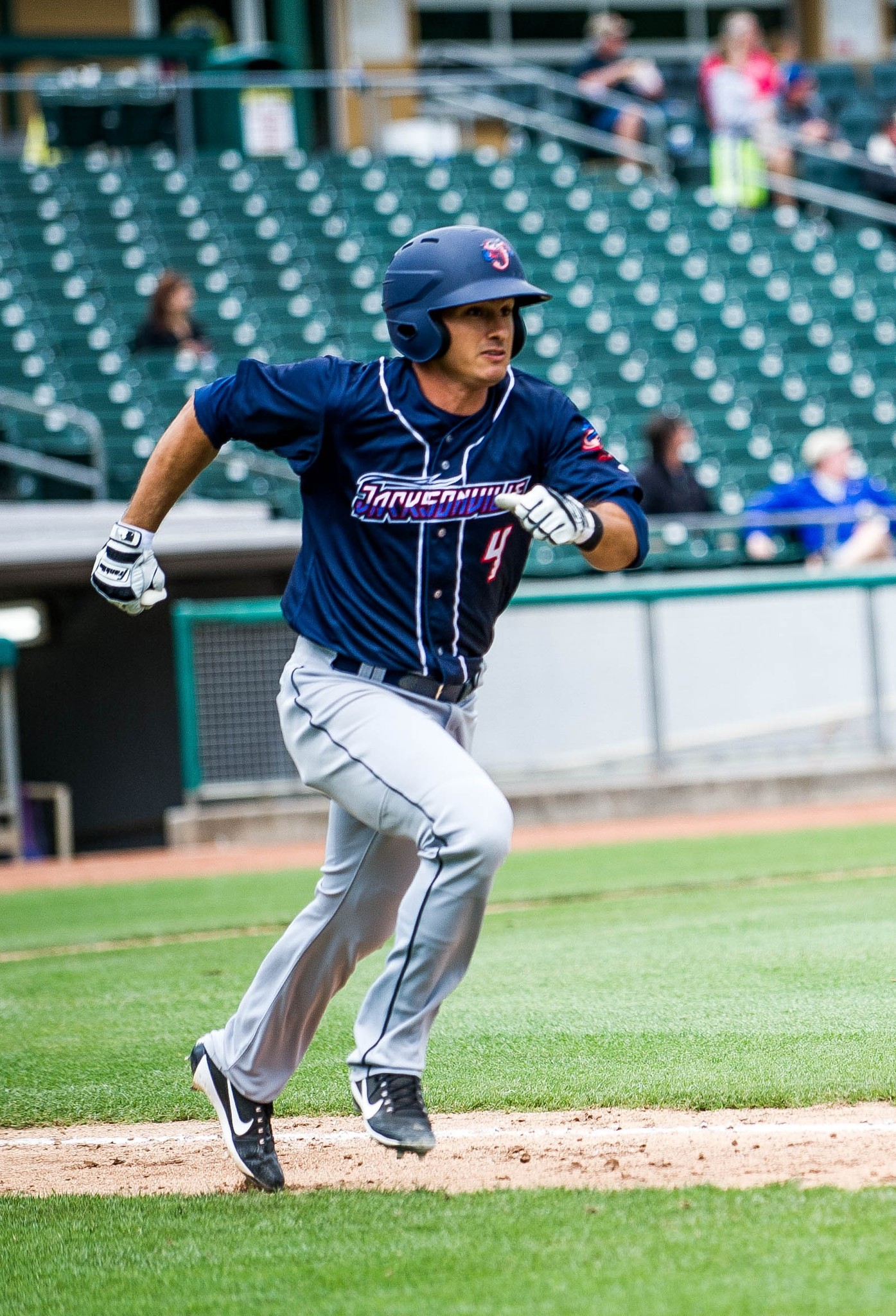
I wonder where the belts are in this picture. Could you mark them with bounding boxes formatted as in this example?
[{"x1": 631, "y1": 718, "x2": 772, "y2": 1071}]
[{"x1": 332, "y1": 653, "x2": 481, "y2": 704}]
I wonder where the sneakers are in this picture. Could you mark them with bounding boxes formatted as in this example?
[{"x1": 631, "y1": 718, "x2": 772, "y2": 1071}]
[
  {"x1": 350, "y1": 1073, "x2": 436, "y2": 1159},
  {"x1": 184, "y1": 1038, "x2": 284, "y2": 1193}
]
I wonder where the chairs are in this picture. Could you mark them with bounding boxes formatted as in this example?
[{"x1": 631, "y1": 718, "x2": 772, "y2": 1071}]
[{"x1": 0, "y1": 140, "x2": 894, "y2": 515}]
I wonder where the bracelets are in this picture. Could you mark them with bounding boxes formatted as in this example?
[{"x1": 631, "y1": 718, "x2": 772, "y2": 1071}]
[{"x1": 572, "y1": 509, "x2": 605, "y2": 552}]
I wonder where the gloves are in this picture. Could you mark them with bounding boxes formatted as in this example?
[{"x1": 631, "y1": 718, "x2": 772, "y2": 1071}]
[
  {"x1": 494, "y1": 484, "x2": 595, "y2": 546},
  {"x1": 91, "y1": 522, "x2": 167, "y2": 617}
]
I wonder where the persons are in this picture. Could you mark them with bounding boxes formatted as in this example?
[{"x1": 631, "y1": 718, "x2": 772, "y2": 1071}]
[
  {"x1": 777, "y1": 60, "x2": 832, "y2": 141},
  {"x1": 702, "y1": 12, "x2": 796, "y2": 205},
  {"x1": 128, "y1": 269, "x2": 210, "y2": 353},
  {"x1": 636, "y1": 411, "x2": 716, "y2": 516},
  {"x1": 740, "y1": 426, "x2": 896, "y2": 570},
  {"x1": 568, "y1": 14, "x2": 662, "y2": 166},
  {"x1": 867, "y1": 118, "x2": 896, "y2": 168},
  {"x1": 90, "y1": 224, "x2": 650, "y2": 1193}
]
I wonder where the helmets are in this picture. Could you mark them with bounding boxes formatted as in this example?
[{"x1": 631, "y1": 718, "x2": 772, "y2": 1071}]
[{"x1": 381, "y1": 224, "x2": 553, "y2": 363}]
